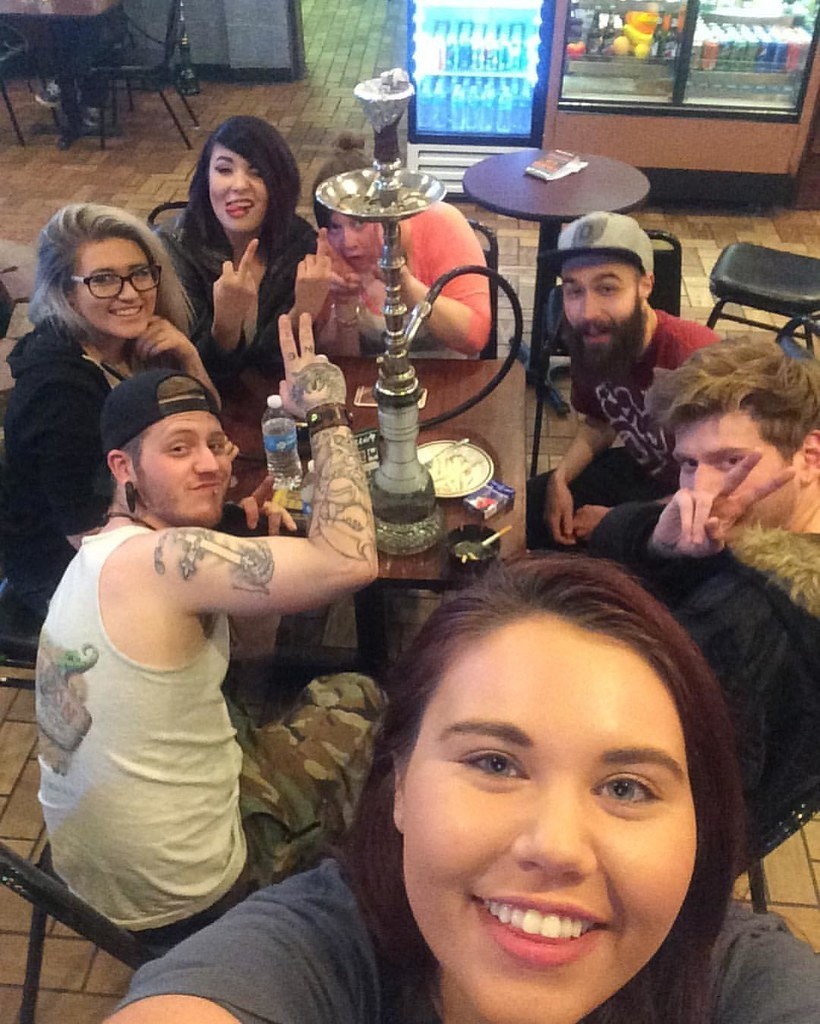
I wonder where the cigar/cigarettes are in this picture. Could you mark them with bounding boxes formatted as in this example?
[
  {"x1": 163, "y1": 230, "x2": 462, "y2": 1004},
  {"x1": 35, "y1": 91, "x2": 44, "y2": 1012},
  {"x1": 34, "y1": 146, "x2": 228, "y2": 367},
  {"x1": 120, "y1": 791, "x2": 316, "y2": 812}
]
[{"x1": 480, "y1": 524, "x2": 512, "y2": 548}]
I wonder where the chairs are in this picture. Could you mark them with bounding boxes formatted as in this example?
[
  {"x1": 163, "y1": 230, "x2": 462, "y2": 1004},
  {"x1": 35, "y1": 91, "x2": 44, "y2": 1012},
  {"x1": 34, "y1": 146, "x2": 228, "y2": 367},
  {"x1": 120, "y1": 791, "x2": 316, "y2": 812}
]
[
  {"x1": 0, "y1": 573, "x2": 54, "y2": 690},
  {"x1": 144, "y1": 202, "x2": 189, "y2": 250},
  {"x1": 0, "y1": 828, "x2": 261, "y2": 1024},
  {"x1": 88, "y1": 0, "x2": 201, "y2": 149},
  {"x1": 457, "y1": 221, "x2": 499, "y2": 361},
  {"x1": 706, "y1": 242, "x2": 820, "y2": 354},
  {"x1": 0, "y1": 20, "x2": 60, "y2": 147},
  {"x1": 529, "y1": 230, "x2": 682, "y2": 486},
  {"x1": 725, "y1": 776, "x2": 820, "y2": 916}
]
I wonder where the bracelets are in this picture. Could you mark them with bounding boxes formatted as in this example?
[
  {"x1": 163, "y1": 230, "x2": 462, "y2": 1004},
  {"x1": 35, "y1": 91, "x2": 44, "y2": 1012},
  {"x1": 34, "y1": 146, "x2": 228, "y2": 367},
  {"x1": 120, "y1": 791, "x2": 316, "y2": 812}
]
[
  {"x1": 330, "y1": 303, "x2": 360, "y2": 327},
  {"x1": 306, "y1": 403, "x2": 354, "y2": 432}
]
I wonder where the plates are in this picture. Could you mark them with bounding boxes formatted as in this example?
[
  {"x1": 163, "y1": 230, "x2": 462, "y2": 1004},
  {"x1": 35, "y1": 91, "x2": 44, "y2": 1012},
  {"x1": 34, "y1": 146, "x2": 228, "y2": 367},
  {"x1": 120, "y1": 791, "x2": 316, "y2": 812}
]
[{"x1": 416, "y1": 439, "x2": 494, "y2": 498}]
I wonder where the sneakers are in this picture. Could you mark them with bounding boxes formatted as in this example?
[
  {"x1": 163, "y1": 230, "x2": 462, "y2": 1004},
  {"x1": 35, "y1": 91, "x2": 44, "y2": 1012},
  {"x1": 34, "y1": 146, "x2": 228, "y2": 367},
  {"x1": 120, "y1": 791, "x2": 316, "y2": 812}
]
[
  {"x1": 78, "y1": 98, "x2": 99, "y2": 126},
  {"x1": 34, "y1": 80, "x2": 61, "y2": 108}
]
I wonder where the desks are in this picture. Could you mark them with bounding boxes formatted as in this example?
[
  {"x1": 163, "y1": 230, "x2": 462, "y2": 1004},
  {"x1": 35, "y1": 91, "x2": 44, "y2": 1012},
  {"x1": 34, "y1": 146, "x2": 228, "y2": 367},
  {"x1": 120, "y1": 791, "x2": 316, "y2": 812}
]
[
  {"x1": 0, "y1": 0, "x2": 134, "y2": 149},
  {"x1": 102, "y1": 351, "x2": 527, "y2": 666},
  {"x1": 0, "y1": 0, "x2": 121, "y2": 150},
  {"x1": 462, "y1": 149, "x2": 652, "y2": 415}
]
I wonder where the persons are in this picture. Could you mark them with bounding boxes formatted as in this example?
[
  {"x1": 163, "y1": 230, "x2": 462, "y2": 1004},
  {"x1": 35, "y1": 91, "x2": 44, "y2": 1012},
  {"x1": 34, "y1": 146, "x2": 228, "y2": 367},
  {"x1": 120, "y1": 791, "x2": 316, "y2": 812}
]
[
  {"x1": 1, "y1": 202, "x2": 222, "y2": 615},
  {"x1": 35, "y1": 309, "x2": 387, "y2": 950},
  {"x1": 101, "y1": 553, "x2": 820, "y2": 1024},
  {"x1": 524, "y1": 211, "x2": 721, "y2": 557},
  {"x1": 312, "y1": 132, "x2": 492, "y2": 357},
  {"x1": 159, "y1": 114, "x2": 317, "y2": 372},
  {"x1": 35, "y1": 0, "x2": 128, "y2": 128},
  {"x1": 588, "y1": 339, "x2": 820, "y2": 849}
]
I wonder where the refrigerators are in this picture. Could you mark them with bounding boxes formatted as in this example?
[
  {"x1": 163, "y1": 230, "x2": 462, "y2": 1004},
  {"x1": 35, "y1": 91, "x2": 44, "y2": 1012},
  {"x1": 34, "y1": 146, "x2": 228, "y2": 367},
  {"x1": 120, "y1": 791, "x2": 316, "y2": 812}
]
[{"x1": 405, "y1": 0, "x2": 820, "y2": 200}]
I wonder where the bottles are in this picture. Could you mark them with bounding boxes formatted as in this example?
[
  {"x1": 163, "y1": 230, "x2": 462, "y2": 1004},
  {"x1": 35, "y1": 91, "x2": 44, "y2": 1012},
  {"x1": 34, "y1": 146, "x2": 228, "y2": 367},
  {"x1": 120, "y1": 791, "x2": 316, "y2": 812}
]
[
  {"x1": 177, "y1": 38, "x2": 200, "y2": 96},
  {"x1": 301, "y1": 459, "x2": 317, "y2": 536},
  {"x1": 420, "y1": 22, "x2": 538, "y2": 135},
  {"x1": 586, "y1": 4, "x2": 676, "y2": 65},
  {"x1": 260, "y1": 394, "x2": 304, "y2": 490}
]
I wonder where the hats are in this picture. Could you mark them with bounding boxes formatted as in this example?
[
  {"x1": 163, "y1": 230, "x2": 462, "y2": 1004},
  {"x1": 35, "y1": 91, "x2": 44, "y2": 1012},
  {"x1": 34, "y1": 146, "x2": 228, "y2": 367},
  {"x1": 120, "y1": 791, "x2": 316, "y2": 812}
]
[
  {"x1": 537, "y1": 212, "x2": 653, "y2": 277},
  {"x1": 98, "y1": 367, "x2": 220, "y2": 457}
]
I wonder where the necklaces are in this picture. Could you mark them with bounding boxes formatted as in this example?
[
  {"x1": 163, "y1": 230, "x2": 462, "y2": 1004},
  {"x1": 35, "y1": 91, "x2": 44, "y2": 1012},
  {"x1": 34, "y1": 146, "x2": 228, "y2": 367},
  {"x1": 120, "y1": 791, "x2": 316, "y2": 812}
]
[{"x1": 102, "y1": 513, "x2": 157, "y2": 532}]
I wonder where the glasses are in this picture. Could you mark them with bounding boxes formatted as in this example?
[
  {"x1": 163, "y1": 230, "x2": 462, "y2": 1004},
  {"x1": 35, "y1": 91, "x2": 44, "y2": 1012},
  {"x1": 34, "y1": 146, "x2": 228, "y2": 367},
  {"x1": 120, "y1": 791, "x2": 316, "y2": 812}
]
[{"x1": 69, "y1": 265, "x2": 161, "y2": 298}]
